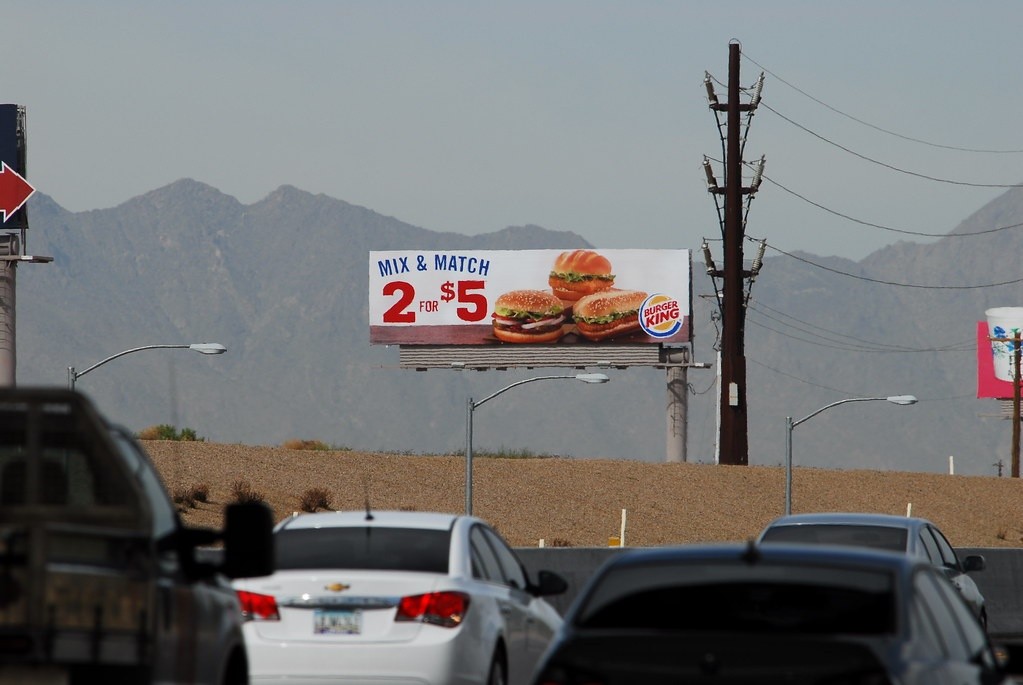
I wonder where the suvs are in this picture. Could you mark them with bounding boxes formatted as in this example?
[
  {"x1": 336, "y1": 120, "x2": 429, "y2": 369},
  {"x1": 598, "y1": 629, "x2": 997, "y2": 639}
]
[{"x1": 0, "y1": 385, "x2": 275, "y2": 685}]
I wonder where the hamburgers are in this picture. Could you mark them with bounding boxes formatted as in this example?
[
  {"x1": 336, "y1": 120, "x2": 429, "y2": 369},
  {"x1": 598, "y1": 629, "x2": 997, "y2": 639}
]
[
  {"x1": 548, "y1": 249, "x2": 616, "y2": 300},
  {"x1": 492, "y1": 291, "x2": 565, "y2": 343},
  {"x1": 574, "y1": 292, "x2": 649, "y2": 339}
]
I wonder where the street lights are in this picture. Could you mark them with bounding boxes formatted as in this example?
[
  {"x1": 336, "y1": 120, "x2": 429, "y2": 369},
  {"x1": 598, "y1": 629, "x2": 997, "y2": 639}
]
[
  {"x1": 68, "y1": 343, "x2": 227, "y2": 391},
  {"x1": 784, "y1": 395, "x2": 918, "y2": 513},
  {"x1": 465, "y1": 373, "x2": 611, "y2": 517}
]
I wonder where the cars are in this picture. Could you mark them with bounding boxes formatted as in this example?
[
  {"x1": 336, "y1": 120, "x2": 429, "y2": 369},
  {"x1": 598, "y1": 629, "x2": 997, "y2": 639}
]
[
  {"x1": 531, "y1": 534, "x2": 1004, "y2": 685},
  {"x1": 228, "y1": 477, "x2": 571, "y2": 685}
]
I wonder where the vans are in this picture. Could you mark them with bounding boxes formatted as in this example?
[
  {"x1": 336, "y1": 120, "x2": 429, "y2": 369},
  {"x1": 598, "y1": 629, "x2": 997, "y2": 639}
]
[{"x1": 752, "y1": 512, "x2": 990, "y2": 633}]
